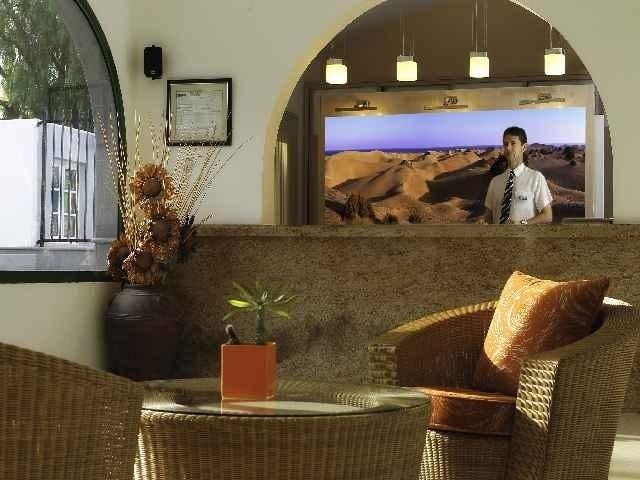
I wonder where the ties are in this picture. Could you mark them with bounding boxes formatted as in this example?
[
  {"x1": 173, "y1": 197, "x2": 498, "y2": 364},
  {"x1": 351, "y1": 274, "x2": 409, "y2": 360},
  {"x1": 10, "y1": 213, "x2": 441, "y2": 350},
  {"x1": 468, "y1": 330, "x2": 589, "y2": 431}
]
[{"x1": 499, "y1": 171, "x2": 513, "y2": 224}]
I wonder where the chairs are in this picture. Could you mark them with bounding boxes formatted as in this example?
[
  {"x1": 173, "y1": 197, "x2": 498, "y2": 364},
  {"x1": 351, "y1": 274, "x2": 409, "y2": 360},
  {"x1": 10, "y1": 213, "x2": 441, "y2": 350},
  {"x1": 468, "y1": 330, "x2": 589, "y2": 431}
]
[
  {"x1": 368, "y1": 297, "x2": 639, "y2": 480},
  {"x1": 0, "y1": 342, "x2": 145, "y2": 480}
]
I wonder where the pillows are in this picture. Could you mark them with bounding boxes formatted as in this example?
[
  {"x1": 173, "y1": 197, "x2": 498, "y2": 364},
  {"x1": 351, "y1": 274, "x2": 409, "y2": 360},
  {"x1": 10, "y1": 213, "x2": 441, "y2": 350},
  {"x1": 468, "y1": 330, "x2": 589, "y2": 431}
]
[{"x1": 468, "y1": 268, "x2": 609, "y2": 396}]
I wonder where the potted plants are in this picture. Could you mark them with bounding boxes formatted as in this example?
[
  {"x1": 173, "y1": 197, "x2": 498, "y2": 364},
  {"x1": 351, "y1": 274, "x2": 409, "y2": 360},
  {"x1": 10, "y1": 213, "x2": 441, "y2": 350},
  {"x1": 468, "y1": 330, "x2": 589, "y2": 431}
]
[{"x1": 220, "y1": 279, "x2": 297, "y2": 401}]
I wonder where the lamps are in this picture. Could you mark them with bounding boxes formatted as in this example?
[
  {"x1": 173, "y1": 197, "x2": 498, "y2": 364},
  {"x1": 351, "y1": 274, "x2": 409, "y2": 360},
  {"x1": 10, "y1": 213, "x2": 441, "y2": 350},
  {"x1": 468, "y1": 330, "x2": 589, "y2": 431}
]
[
  {"x1": 543, "y1": 24, "x2": 566, "y2": 75},
  {"x1": 469, "y1": 0, "x2": 491, "y2": 79},
  {"x1": 396, "y1": 0, "x2": 418, "y2": 83},
  {"x1": 325, "y1": 45, "x2": 348, "y2": 84}
]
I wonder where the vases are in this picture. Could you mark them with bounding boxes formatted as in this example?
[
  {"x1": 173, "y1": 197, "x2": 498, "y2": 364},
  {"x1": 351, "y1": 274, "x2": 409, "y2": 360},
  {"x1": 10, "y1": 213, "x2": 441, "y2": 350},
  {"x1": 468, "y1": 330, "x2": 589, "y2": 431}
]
[{"x1": 103, "y1": 283, "x2": 194, "y2": 381}]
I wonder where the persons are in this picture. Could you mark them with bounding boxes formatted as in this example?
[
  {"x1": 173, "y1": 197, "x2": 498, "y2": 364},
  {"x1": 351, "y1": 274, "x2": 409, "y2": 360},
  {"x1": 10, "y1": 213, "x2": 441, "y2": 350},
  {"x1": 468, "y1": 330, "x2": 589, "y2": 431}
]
[{"x1": 482, "y1": 126, "x2": 554, "y2": 224}]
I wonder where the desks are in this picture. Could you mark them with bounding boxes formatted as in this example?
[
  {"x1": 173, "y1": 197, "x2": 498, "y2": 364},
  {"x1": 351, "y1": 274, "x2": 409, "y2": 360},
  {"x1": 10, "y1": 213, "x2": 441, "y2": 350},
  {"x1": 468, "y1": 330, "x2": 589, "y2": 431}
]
[{"x1": 133, "y1": 375, "x2": 434, "y2": 480}]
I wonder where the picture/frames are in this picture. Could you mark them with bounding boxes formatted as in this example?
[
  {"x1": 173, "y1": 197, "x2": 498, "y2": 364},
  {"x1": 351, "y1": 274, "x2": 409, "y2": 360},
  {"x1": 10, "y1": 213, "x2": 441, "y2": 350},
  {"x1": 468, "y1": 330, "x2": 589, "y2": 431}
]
[
  {"x1": 164, "y1": 77, "x2": 233, "y2": 147},
  {"x1": 301, "y1": 78, "x2": 605, "y2": 225}
]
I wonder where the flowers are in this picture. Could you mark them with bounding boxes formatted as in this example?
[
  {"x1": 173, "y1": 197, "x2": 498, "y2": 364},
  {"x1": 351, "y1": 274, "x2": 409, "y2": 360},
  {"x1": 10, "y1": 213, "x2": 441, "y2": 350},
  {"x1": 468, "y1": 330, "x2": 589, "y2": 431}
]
[{"x1": 91, "y1": 103, "x2": 256, "y2": 286}]
